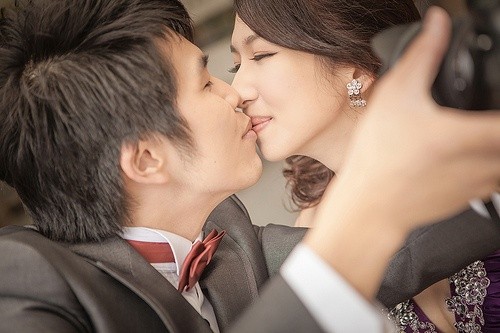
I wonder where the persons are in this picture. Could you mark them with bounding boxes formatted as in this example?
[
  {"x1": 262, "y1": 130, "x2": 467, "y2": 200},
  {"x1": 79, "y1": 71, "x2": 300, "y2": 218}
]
[
  {"x1": 0, "y1": 0, "x2": 500, "y2": 333},
  {"x1": 226, "y1": 0, "x2": 500, "y2": 333}
]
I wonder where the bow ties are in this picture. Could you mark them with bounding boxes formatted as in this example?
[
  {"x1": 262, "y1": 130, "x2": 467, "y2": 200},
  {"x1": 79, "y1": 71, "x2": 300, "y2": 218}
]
[{"x1": 125, "y1": 228, "x2": 227, "y2": 295}]
